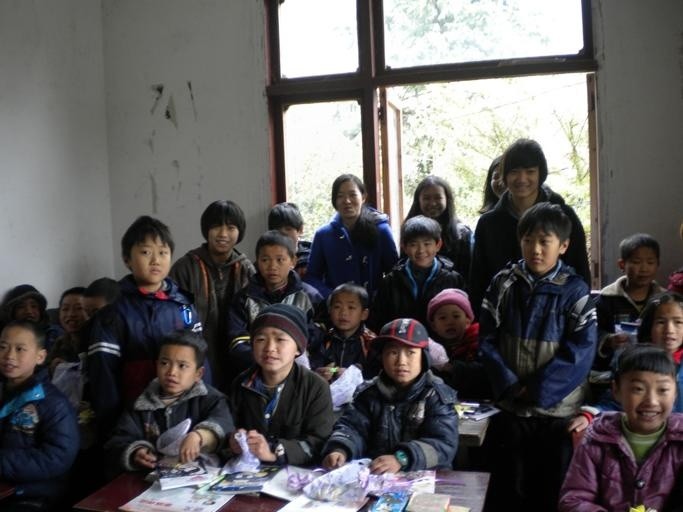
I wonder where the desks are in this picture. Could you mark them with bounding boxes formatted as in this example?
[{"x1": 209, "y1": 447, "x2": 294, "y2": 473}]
[
  {"x1": 455, "y1": 402, "x2": 493, "y2": 448},
  {"x1": 71, "y1": 467, "x2": 492, "y2": 511}
]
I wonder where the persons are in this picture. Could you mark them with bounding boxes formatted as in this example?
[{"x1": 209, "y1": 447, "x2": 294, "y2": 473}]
[
  {"x1": 562, "y1": 341, "x2": 683, "y2": 512},
  {"x1": 319, "y1": 320, "x2": 460, "y2": 475},
  {"x1": 87, "y1": 214, "x2": 212, "y2": 435},
  {"x1": 304, "y1": 284, "x2": 375, "y2": 389},
  {"x1": 587, "y1": 230, "x2": 671, "y2": 355},
  {"x1": 470, "y1": 137, "x2": 589, "y2": 280},
  {"x1": 309, "y1": 176, "x2": 397, "y2": 290},
  {"x1": 85, "y1": 278, "x2": 119, "y2": 318},
  {"x1": 480, "y1": 155, "x2": 508, "y2": 206},
  {"x1": 108, "y1": 337, "x2": 232, "y2": 469},
  {"x1": 369, "y1": 216, "x2": 463, "y2": 312},
  {"x1": 171, "y1": 198, "x2": 255, "y2": 328},
  {"x1": 426, "y1": 288, "x2": 486, "y2": 396},
  {"x1": 226, "y1": 302, "x2": 332, "y2": 464},
  {"x1": 400, "y1": 177, "x2": 472, "y2": 269},
  {"x1": 620, "y1": 295, "x2": 681, "y2": 416},
  {"x1": 258, "y1": 203, "x2": 311, "y2": 284},
  {"x1": 228, "y1": 230, "x2": 318, "y2": 365},
  {"x1": 0, "y1": 323, "x2": 80, "y2": 511},
  {"x1": 6, "y1": 283, "x2": 65, "y2": 363},
  {"x1": 49, "y1": 286, "x2": 98, "y2": 397},
  {"x1": 476, "y1": 206, "x2": 596, "y2": 511}
]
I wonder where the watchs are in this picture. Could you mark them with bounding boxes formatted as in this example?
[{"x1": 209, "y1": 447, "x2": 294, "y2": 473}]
[{"x1": 395, "y1": 450, "x2": 410, "y2": 472}]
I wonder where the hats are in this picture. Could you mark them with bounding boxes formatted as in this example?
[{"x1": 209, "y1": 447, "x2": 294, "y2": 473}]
[
  {"x1": 2, "y1": 283, "x2": 48, "y2": 317},
  {"x1": 426, "y1": 287, "x2": 474, "y2": 324},
  {"x1": 368, "y1": 318, "x2": 429, "y2": 349},
  {"x1": 249, "y1": 302, "x2": 309, "y2": 358}
]
